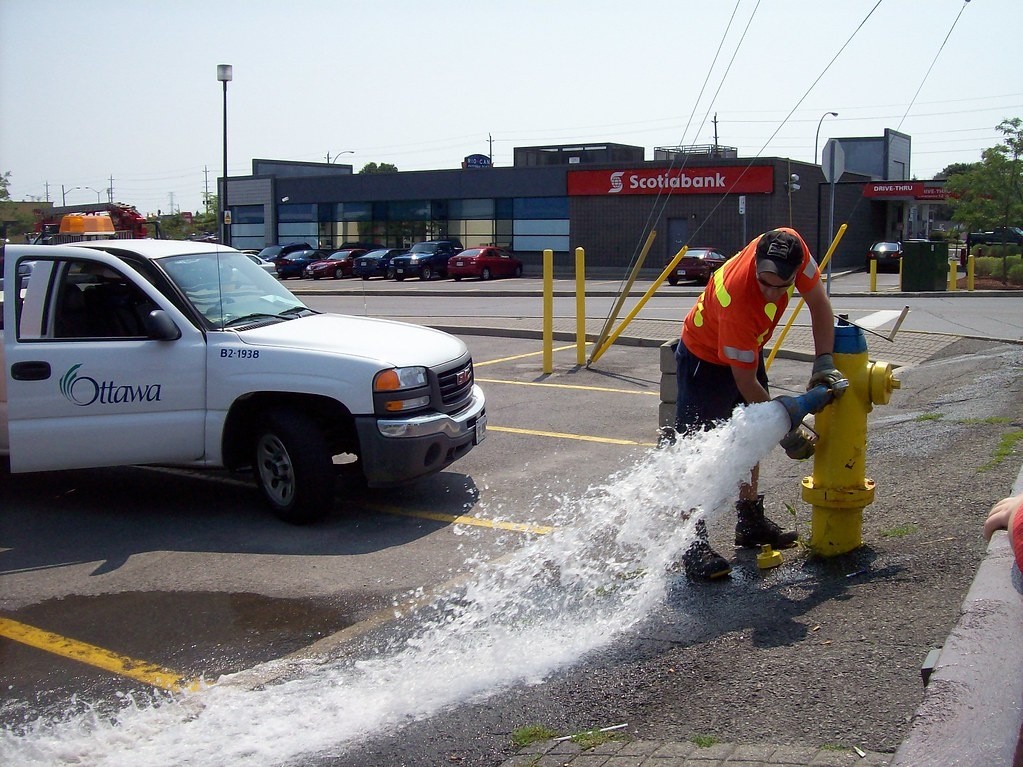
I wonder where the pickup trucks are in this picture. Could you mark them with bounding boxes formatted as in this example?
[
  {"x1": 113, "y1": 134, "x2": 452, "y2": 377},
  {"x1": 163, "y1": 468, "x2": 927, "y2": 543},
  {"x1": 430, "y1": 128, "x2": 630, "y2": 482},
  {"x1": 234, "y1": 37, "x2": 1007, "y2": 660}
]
[
  {"x1": 969, "y1": 226, "x2": 1023, "y2": 246},
  {"x1": 0, "y1": 238, "x2": 488, "y2": 528}
]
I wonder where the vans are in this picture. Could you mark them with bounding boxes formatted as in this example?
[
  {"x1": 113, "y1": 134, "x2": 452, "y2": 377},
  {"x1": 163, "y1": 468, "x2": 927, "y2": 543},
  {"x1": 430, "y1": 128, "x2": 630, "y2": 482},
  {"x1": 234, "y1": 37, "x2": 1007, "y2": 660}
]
[{"x1": 337, "y1": 243, "x2": 384, "y2": 251}]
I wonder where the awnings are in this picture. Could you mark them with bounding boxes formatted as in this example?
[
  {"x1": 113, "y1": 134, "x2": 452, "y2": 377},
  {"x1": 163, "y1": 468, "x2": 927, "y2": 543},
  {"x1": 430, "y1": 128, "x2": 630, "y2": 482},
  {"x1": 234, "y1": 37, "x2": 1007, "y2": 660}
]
[{"x1": 44, "y1": 222, "x2": 60, "y2": 226}]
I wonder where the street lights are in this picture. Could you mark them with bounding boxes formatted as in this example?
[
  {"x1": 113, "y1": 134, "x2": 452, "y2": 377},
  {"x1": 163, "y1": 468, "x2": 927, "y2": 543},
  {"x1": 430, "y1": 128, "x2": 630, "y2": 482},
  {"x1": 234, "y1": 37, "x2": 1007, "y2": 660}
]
[
  {"x1": 60, "y1": 184, "x2": 81, "y2": 210},
  {"x1": 814, "y1": 112, "x2": 839, "y2": 165},
  {"x1": 217, "y1": 64, "x2": 233, "y2": 249},
  {"x1": 83, "y1": 186, "x2": 108, "y2": 203},
  {"x1": 25, "y1": 193, "x2": 50, "y2": 202}
]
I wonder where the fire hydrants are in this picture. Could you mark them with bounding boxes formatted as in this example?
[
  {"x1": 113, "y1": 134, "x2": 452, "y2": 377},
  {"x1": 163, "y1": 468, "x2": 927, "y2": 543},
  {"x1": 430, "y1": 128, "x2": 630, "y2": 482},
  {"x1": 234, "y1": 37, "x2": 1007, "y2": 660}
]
[{"x1": 800, "y1": 312, "x2": 903, "y2": 559}]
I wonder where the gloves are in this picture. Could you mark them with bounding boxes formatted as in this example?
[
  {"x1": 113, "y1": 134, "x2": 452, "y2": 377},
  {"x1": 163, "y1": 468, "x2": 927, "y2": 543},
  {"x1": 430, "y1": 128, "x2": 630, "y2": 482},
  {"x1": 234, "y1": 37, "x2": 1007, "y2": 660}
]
[
  {"x1": 780, "y1": 427, "x2": 816, "y2": 459},
  {"x1": 806, "y1": 353, "x2": 846, "y2": 401}
]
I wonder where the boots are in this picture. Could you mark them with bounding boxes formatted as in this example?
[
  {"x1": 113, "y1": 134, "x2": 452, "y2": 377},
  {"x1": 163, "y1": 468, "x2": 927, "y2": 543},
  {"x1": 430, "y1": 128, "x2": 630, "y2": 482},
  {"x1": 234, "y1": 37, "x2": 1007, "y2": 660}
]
[
  {"x1": 682, "y1": 519, "x2": 733, "y2": 579},
  {"x1": 734, "y1": 494, "x2": 798, "y2": 547}
]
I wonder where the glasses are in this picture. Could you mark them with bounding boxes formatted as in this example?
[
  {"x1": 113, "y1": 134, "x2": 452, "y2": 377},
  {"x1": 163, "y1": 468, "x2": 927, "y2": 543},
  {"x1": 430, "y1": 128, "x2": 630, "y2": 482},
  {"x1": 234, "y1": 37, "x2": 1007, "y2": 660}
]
[{"x1": 758, "y1": 273, "x2": 796, "y2": 288}]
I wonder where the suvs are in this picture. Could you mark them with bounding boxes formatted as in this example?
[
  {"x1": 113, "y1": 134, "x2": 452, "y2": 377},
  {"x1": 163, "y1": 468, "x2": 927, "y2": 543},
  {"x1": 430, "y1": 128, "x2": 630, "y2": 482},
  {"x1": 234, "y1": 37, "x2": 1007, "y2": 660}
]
[
  {"x1": 257, "y1": 241, "x2": 314, "y2": 262},
  {"x1": 388, "y1": 241, "x2": 456, "y2": 283}
]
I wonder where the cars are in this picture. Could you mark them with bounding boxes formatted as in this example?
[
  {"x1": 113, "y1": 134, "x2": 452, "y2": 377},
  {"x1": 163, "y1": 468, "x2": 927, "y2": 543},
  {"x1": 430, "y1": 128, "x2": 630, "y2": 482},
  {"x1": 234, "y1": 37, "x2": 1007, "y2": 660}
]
[
  {"x1": 447, "y1": 247, "x2": 523, "y2": 280},
  {"x1": 240, "y1": 249, "x2": 261, "y2": 261},
  {"x1": 866, "y1": 242, "x2": 902, "y2": 273},
  {"x1": 303, "y1": 249, "x2": 368, "y2": 281},
  {"x1": 229, "y1": 253, "x2": 280, "y2": 282},
  {"x1": 352, "y1": 247, "x2": 411, "y2": 280},
  {"x1": 663, "y1": 247, "x2": 730, "y2": 285},
  {"x1": 274, "y1": 248, "x2": 334, "y2": 280}
]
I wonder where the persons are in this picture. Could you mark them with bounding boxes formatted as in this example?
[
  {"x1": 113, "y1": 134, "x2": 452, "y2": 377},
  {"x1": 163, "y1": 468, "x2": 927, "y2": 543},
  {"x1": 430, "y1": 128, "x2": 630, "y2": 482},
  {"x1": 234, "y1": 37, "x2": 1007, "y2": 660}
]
[
  {"x1": 41, "y1": 225, "x2": 53, "y2": 244},
  {"x1": 673, "y1": 227, "x2": 850, "y2": 582},
  {"x1": 26, "y1": 234, "x2": 38, "y2": 244},
  {"x1": 155, "y1": 210, "x2": 164, "y2": 239},
  {"x1": 225, "y1": 212, "x2": 230, "y2": 218},
  {"x1": 115, "y1": 255, "x2": 161, "y2": 334},
  {"x1": 438, "y1": 228, "x2": 446, "y2": 241}
]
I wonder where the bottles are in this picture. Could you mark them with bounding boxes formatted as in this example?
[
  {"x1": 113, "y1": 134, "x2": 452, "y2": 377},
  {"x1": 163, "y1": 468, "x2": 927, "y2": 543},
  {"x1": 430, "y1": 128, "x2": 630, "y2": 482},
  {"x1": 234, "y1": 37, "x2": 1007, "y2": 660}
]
[{"x1": 226, "y1": 212, "x2": 230, "y2": 222}]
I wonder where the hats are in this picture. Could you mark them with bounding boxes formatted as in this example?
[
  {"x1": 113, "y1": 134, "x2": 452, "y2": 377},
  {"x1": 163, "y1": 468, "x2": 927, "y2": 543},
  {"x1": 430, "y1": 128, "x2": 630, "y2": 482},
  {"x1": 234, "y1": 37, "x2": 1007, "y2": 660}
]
[{"x1": 756, "y1": 230, "x2": 803, "y2": 281}]
